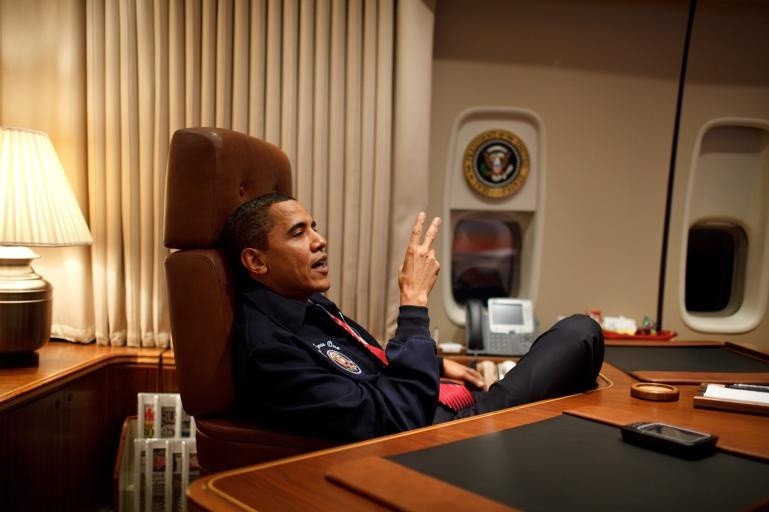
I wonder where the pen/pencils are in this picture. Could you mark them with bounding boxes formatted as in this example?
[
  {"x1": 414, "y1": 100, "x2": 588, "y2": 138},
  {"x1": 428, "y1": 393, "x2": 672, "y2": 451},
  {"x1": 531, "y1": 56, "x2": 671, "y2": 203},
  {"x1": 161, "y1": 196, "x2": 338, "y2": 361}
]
[{"x1": 725, "y1": 383, "x2": 769, "y2": 392}]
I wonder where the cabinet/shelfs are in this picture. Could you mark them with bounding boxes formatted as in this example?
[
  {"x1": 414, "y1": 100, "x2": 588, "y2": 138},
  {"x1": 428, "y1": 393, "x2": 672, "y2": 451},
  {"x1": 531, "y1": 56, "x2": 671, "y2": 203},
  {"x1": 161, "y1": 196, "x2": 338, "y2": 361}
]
[{"x1": 0, "y1": 338, "x2": 169, "y2": 512}]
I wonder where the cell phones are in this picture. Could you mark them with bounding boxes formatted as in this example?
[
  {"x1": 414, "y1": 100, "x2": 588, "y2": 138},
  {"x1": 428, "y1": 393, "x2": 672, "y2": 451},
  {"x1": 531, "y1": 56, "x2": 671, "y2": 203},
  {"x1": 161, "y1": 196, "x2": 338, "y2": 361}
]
[{"x1": 620, "y1": 420, "x2": 717, "y2": 462}]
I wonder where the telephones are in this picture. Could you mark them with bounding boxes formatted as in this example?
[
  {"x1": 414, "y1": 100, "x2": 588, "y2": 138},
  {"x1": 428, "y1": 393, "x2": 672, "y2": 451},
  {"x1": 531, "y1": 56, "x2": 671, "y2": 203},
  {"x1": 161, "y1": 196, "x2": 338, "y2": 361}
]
[{"x1": 465, "y1": 297, "x2": 539, "y2": 356}]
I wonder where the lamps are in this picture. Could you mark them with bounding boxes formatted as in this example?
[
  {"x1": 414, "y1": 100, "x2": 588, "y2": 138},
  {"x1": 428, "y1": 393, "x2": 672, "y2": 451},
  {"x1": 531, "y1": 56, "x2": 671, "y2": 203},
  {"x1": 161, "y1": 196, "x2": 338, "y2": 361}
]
[{"x1": 0, "y1": 123, "x2": 94, "y2": 371}]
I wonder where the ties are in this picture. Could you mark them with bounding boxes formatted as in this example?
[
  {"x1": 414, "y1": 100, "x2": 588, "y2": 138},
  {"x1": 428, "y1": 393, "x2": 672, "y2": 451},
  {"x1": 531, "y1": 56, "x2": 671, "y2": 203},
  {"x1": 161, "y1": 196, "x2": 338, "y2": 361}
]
[{"x1": 304, "y1": 298, "x2": 477, "y2": 412}]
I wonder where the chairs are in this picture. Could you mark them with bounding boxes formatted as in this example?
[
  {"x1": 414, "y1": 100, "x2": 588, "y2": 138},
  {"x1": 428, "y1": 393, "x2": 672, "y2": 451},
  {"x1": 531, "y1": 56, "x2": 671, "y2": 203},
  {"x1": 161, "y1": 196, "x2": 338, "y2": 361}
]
[{"x1": 163, "y1": 121, "x2": 323, "y2": 471}]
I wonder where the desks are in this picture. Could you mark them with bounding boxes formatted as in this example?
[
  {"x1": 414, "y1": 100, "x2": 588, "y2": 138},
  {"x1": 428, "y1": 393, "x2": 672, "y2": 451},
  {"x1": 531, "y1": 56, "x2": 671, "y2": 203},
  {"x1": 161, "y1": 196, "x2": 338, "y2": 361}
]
[{"x1": 182, "y1": 337, "x2": 768, "y2": 509}]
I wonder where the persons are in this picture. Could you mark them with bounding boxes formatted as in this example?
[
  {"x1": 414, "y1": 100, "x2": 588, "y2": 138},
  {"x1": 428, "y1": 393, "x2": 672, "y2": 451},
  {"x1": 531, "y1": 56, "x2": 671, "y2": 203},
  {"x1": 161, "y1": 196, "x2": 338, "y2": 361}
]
[{"x1": 228, "y1": 190, "x2": 606, "y2": 446}]
